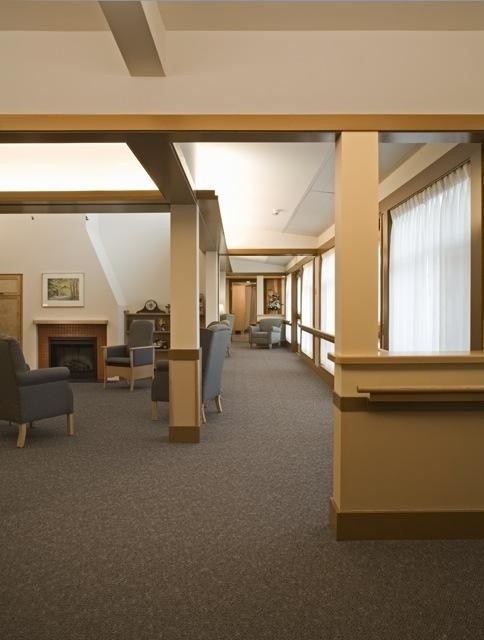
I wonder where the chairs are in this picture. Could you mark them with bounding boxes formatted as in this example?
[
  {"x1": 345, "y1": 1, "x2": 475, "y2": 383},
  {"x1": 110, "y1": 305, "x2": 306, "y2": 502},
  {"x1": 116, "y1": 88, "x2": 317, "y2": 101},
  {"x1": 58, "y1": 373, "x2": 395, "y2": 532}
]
[
  {"x1": 248, "y1": 318, "x2": 285, "y2": 349},
  {"x1": 0, "y1": 335, "x2": 74, "y2": 448},
  {"x1": 100, "y1": 314, "x2": 235, "y2": 422}
]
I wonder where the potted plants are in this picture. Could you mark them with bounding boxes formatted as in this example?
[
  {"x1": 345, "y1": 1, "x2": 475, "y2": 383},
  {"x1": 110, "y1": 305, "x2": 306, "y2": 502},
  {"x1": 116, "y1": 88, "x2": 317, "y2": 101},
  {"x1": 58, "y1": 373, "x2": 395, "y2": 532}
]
[{"x1": 266, "y1": 295, "x2": 281, "y2": 314}]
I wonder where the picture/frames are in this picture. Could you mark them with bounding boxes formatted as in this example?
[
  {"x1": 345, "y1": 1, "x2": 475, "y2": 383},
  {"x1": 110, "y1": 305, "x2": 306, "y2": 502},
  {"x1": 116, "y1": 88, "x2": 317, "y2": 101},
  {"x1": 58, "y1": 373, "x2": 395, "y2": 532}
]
[{"x1": 42, "y1": 273, "x2": 84, "y2": 307}]
